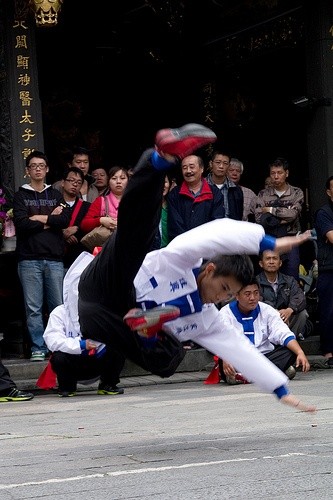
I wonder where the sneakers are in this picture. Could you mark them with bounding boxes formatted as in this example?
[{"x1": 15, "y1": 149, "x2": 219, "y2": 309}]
[
  {"x1": 57, "y1": 391, "x2": 77, "y2": 397},
  {"x1": 123, "y1": 305, "x2": 180, "y2": 338},
  {"x1": 98, "y1": 381, "x2": 124, "y2": 394},
  {"x1": 154, "y1": 123, "x2": 217, "y2": 162},
  {"x1": 30, "y1": 351, "x2": 45, "y2": 360},
  {"x1": 0, "y1": 386, "x2": 34, "y2": 402}
]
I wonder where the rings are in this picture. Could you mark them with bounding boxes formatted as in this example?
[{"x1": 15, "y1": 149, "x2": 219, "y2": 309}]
[{"x1": 282, "y1": 317, "x2": 285, "y2": 320}]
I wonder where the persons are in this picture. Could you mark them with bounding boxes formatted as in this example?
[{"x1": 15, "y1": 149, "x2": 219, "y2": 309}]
[
  {"x1": 0, "y1": 359, "x2": 33, "y2": 401},
  {"x1": 219, "y1": 278, "x2": 309, "y2": 385},
  {"x1": 256, "y1": 249, "x2": 309, "y2": 337},
  {"x1": 43, "y1": 305, "x2": 125, "y2": 398},
  {"x1": 314, "y1": 176, "x2": 333, "y2": 360},
  {"x1": 52, "y1": 147, "x2": 134, "y2": 268},
  {"x1": 14, "y1": 150, "x2": 73, "y2": 361},
  {"x1": 157, "y1": 153, "x2": 309, "y2": 350},
  {"x1": 78, "y1": 124, "x2": 317, "y2": 413}
]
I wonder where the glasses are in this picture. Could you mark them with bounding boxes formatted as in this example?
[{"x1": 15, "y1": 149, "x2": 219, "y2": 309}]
[
  {"x1": 65, "y1": 179, "x2": 82, "y2": 186},
  {"x1": 27, "y1": 163, "x2": 47, "y2": 170}
]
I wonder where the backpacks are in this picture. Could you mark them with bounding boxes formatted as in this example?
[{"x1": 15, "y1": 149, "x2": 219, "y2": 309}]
[{"x1": 259, "y1": 199, "x2": 293, "y2": 238}]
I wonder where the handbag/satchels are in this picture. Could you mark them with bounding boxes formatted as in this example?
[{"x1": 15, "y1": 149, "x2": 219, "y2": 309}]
[{"x1": 80, "y1": 196, "x2": 113, "y2": 251}]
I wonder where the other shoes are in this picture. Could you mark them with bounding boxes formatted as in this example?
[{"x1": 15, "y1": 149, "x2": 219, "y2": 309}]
[
  {"x1": 320, "y1": 357, "x2": 333, "y2": 368},
  {"x1": 285, "y1": 365, "x2": 296, "y2": 380},
  {"x1": 226, "y1": 373, "x2": 251, "y2": 384},
  {"x1": 297, "y1": 334, "x2": 304, "y2": 340}
]
